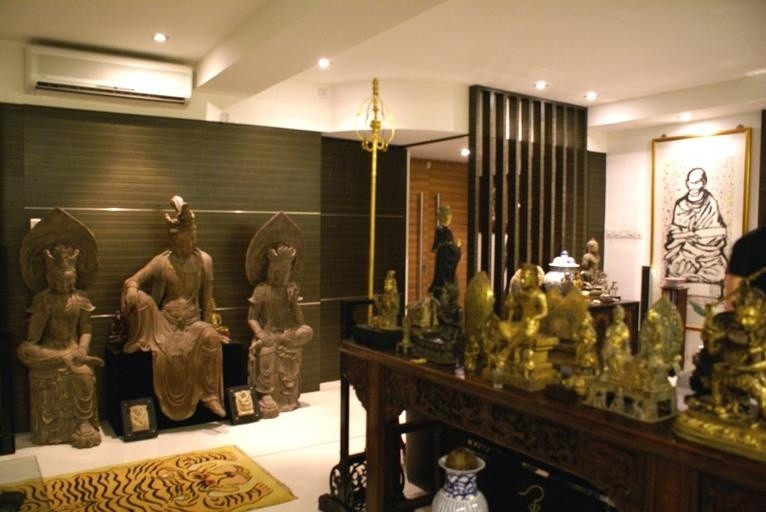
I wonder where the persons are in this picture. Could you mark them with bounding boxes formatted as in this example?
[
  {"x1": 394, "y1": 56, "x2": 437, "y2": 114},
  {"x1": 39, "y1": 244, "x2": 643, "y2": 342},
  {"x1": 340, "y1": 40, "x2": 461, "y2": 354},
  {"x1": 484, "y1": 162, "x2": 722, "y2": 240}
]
[
  {"x1": 239, "y1": 244, "x2": 317, "y2": 418},
  {"x1": 120, "y1": 194, "x2": 232, "y2": 419},
  {"x1": 14, "y1": 239, "x2": 107, "y2": 440},
  {"x1": 660, "y1": 168, "x2": 728, "y2": 282},
  {"x1": 371, "y1": 205, "x2": 766, "y2": 423}
]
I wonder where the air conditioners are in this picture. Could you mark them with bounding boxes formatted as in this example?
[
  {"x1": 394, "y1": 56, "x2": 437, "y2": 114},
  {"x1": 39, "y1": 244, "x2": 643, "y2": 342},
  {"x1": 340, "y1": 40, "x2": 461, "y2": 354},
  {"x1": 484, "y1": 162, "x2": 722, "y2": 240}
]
[{"x1": 20, "y1": 41, "x2": 194, "y2": 111}]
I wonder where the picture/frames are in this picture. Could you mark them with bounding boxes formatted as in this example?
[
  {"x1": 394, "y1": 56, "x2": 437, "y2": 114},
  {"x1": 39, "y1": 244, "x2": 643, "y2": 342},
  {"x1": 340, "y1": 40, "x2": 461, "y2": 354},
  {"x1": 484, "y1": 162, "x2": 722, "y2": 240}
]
[{"x1": 648, "y1": 126, "x2": 751, "y2": 333}]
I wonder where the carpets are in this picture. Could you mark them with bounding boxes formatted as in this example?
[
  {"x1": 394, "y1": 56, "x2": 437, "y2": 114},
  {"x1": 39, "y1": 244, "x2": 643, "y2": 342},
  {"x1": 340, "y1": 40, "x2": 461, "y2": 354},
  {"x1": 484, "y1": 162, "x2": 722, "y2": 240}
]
[{"x1": 0, "y1": 441, "x2": 297, "y2": 511}]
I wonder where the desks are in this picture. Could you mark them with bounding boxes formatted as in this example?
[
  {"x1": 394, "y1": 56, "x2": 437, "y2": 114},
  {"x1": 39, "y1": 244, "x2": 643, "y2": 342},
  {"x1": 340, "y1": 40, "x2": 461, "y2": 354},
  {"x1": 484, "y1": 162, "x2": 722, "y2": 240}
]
[{"x1": 336, "y1": 336, "x2": 765, "y2": 510}]
